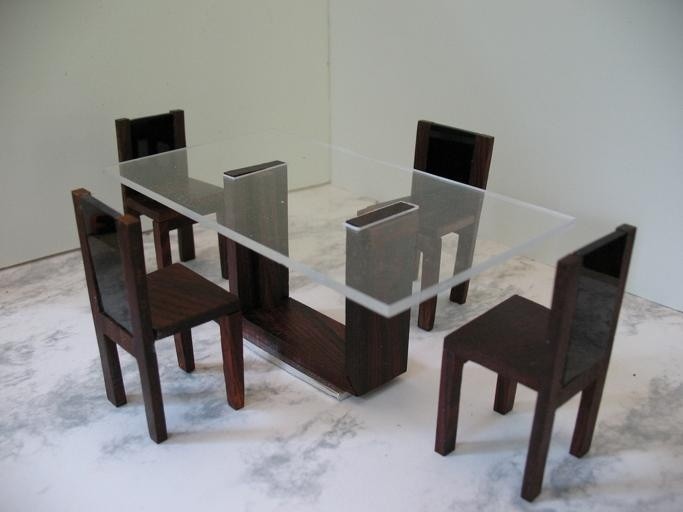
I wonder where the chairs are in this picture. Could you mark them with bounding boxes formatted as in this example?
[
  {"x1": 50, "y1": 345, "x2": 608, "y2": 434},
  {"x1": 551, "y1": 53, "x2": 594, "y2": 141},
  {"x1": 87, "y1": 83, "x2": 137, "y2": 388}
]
[
  {"x1": 434, "y1": 223, "x2": 636, "y2": 503},
  {"x1": 115, "y1": 108, "x2": 230, "y2": 281},
  {"x1": 70, "y1": 188, "x2": 245, "y2": 443},
  {"x1": 355, "y1": 120, "x2": 496, "y2": 331}
]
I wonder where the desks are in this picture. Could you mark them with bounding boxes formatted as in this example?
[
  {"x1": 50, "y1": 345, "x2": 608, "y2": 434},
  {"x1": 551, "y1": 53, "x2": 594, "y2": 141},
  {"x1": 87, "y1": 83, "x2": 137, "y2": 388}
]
[{"x1": 101, "y1": 126, "x2": 575, "y2": 402}]
